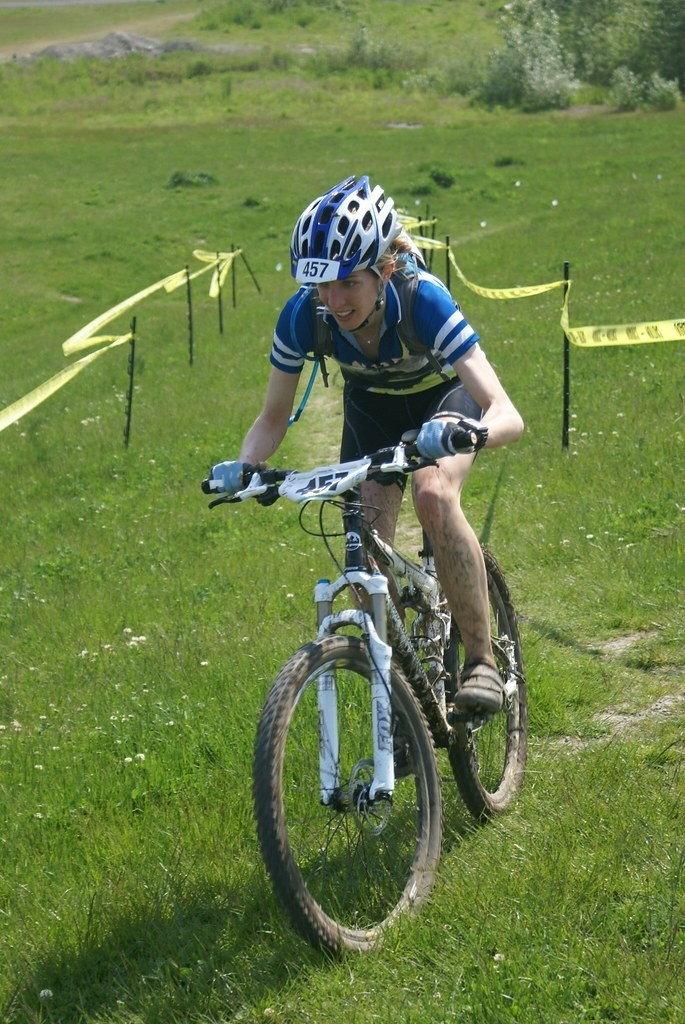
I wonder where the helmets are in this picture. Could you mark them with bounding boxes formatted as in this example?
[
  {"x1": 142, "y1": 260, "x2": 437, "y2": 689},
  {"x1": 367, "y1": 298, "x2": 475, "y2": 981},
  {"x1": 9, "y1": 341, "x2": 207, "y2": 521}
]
[{"x1": 291, "y1": 176, "x2": 402, "y2": 283}]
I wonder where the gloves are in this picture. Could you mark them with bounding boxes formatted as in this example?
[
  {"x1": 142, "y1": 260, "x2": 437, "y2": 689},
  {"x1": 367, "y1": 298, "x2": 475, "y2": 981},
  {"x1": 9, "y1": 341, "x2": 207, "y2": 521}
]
[
  {"x1": 209, "y1": 461, "x2": 256, "y2": 495},
  {"x1": 416, "y1": 419, "x2": 474, "y2": 460}
]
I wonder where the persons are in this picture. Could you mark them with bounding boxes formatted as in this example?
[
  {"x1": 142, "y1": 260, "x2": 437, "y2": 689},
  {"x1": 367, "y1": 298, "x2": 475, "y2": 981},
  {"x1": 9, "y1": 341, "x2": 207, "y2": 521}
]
[{"x1": 206, "y1": 173, "x2": 524, "y2": 779}]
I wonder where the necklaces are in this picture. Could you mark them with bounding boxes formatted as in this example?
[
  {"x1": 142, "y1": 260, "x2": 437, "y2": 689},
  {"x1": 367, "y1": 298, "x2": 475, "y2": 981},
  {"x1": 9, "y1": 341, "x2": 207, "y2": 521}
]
[{"x1": 359, "y1": 329, "x2": 378, "y2": 344}]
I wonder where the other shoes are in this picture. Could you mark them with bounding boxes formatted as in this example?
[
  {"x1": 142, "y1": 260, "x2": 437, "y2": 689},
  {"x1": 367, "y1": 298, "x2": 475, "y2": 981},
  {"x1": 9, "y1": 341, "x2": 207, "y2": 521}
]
[
  {"x1": 454, "y1": 656, "x2": 507, "y2": 715},
  {"x1": 393, "y1": 711, "x2": 416, "y2": 779}
]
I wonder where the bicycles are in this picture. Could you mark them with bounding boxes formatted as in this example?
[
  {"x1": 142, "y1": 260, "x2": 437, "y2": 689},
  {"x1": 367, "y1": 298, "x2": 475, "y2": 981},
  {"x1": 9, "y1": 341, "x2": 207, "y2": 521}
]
[{"x1": 199, "y1": 425, "x2": 534, "y2": 960}]
